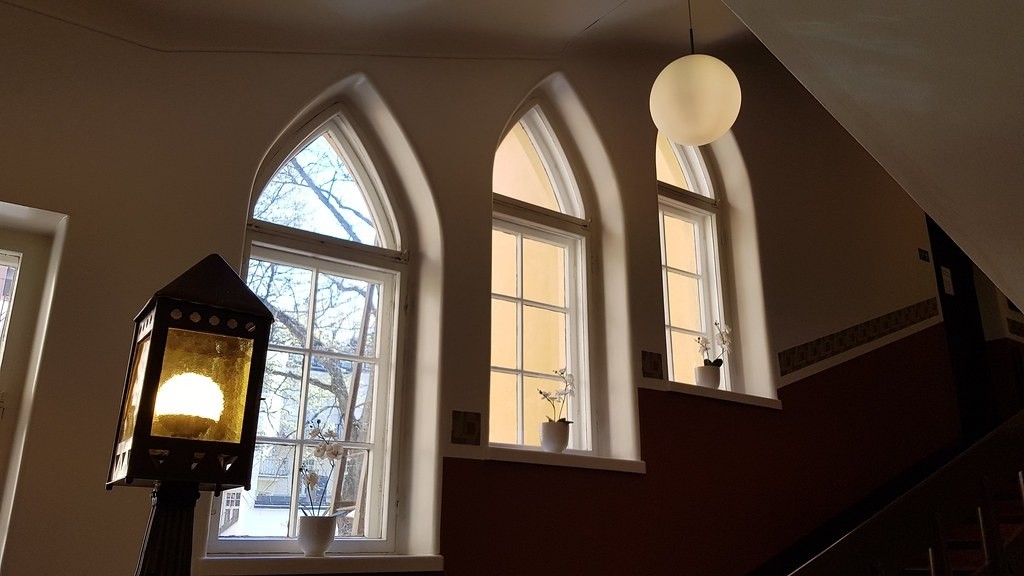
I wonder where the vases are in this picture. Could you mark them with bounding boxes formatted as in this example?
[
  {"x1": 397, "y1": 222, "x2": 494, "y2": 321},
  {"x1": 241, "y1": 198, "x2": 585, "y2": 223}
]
[
  {"x1": 299, "y1": 516, "x2": 336, "y2": 558},
  {"x1": 541, "y1": 421, "x2": 569, "y2": 453},
  {"x1": 698, "y1": 365, "x2": 720, "y2": 389}
]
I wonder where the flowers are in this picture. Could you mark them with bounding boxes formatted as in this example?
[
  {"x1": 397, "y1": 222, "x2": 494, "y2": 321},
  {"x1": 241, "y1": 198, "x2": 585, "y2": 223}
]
[
  {"x1": 296, "y1": 420, "x2": 354, "y2": 517},
  {"x1": 697, "y1": 320, "x2": 733, "y2": 366},
  {"x1": 538, "y1": 365, "x2": 573, "y2": 424}
]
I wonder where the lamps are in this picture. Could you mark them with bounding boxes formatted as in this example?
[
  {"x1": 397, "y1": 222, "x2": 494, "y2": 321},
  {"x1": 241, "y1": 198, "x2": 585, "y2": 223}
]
[
  {"x1": 647, "y1": 2, "x2": 742, "y2": 148},
  {"x1": 106, "y1": 248, "x2": 275, "y2": 576}
]
